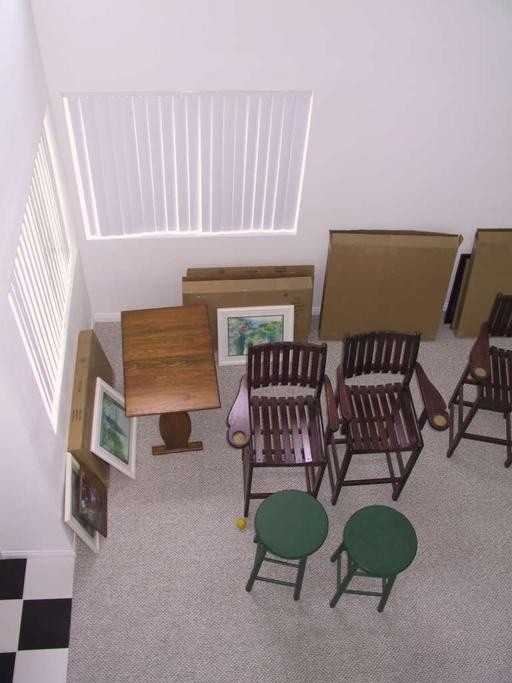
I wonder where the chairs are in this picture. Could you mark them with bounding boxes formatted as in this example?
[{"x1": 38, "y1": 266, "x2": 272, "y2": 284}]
[
  {"x1": 227, "y1": 341, "x2": 339, "y2": 516},
  {"x1": 449, "y1": 291, "x2": 511, "y2": 468},
  {"x1": 315, "y1": 332, "x2": 448, "y2": 504}
]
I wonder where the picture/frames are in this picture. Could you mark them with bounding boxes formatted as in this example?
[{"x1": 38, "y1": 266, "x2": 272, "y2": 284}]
[
  {"x1": 216, "y1": 304, "x2": 296, "y2": 367},
  {"x1": 63, "y1": 374, "x2": 139, "y2": 553}
]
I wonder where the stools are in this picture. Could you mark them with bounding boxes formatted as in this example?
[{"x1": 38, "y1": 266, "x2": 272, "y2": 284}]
[{"x1": 244, "y1": 488, "x2": 419, "y2": 613}]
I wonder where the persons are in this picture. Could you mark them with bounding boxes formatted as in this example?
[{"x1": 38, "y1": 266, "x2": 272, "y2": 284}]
[{"x1": 235, "y1": 325, "x2": 254, "y2": 355}]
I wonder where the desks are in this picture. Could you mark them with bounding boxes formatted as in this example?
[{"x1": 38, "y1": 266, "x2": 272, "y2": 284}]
[{"x1": 120, "y1": 302, "x2": 222, "y2": 456}]
